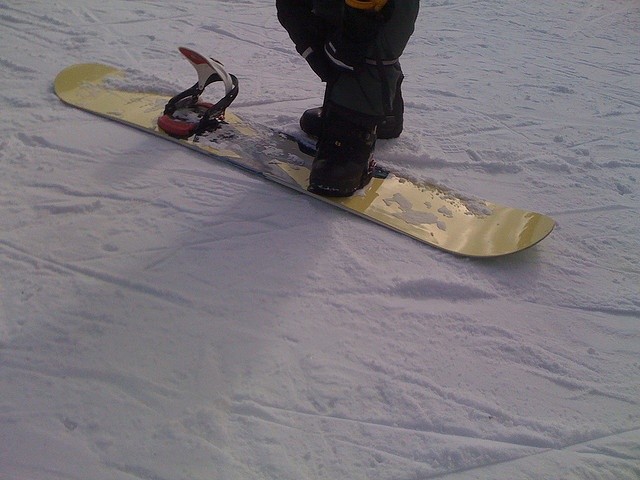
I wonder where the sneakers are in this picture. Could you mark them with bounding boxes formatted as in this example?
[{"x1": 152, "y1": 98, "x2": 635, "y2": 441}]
[
  {"x1": 300, "y1": 103, "x2": 405, "y2": 139},
  {"x1": 307, "y1": 143, "x2": 379, "y2": 198}
]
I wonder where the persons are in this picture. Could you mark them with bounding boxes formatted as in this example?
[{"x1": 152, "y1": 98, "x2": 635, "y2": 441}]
[{"x1": 274, "y1": 1, "x2": 420, "y2": 198}]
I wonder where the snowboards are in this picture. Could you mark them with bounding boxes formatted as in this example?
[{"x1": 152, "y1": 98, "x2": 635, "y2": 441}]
[{"x1": 53, "y1": 45, "x2": 556, "y2": 259}]
